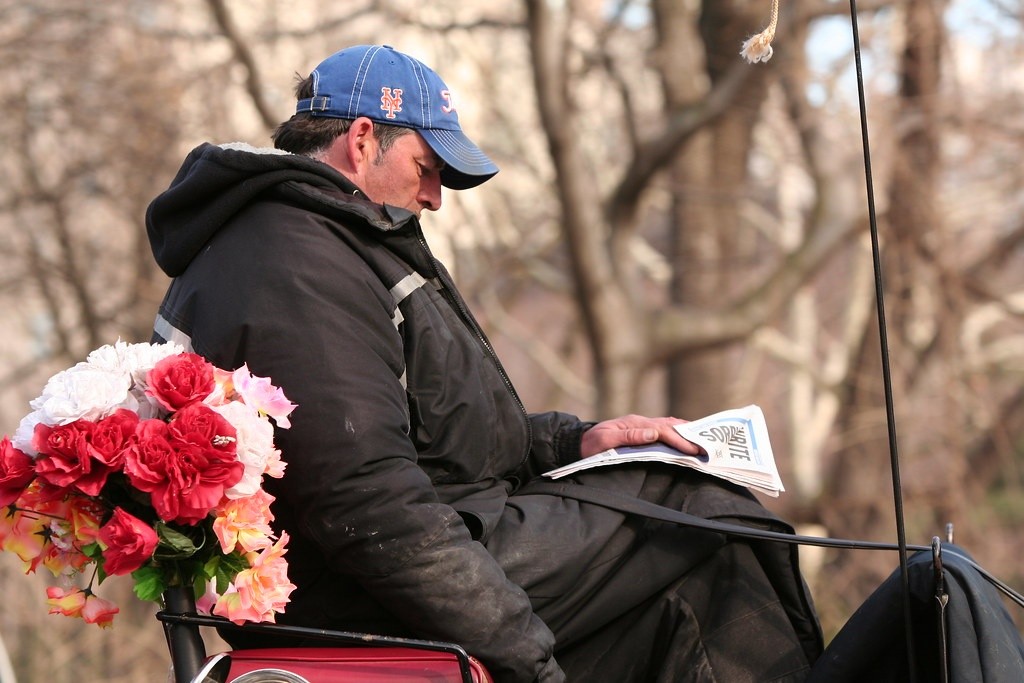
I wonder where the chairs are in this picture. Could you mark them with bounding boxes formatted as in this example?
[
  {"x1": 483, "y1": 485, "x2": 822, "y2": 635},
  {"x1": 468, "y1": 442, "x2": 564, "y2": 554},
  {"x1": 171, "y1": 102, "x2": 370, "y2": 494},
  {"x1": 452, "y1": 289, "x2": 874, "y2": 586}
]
[{"x1": 156, "y1": 577, "x2": 494, "y2": 683}]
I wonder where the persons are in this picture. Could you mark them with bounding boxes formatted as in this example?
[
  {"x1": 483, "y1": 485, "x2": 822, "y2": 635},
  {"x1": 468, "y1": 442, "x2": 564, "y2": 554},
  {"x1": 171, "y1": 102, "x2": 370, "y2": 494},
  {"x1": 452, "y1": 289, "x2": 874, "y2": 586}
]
[{"x1": 146, "y1": 46, "x2": 827, "y2": 683}]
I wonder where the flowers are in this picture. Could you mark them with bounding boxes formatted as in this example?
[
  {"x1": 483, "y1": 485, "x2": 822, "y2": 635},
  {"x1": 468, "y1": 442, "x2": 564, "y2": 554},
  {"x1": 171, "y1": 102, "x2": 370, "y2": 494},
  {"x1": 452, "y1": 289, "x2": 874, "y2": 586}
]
[{"x1": 0, "y1": 335, "x2": 299, "y2": 627}]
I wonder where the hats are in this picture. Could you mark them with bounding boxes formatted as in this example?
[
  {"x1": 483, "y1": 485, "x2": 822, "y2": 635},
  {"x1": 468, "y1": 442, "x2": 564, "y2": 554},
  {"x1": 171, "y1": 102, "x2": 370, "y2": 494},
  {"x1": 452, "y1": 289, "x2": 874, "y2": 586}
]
[{"x1": 297, "y1": 44, "x2": 500, "y2": 190}]
